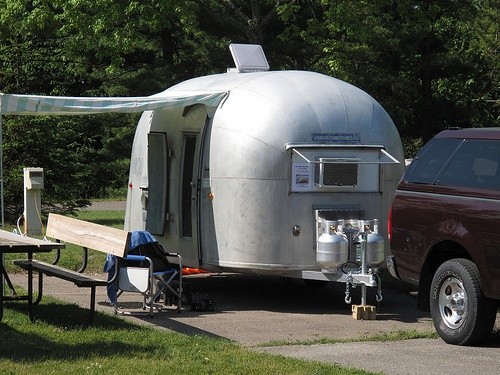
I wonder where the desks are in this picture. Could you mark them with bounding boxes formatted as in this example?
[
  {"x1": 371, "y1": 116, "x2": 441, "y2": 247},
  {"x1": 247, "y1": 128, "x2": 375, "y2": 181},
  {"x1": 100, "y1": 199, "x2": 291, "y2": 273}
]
[{"x1": 0, "y1": 229, "x2": 66, "y2": 323}]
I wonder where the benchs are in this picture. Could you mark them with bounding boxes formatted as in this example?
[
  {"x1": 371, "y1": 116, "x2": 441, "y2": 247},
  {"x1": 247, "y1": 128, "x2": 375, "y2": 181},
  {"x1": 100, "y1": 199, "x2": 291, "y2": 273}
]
[{"x1": 12, "y1": 213, "x2": 128, "y2": 324}]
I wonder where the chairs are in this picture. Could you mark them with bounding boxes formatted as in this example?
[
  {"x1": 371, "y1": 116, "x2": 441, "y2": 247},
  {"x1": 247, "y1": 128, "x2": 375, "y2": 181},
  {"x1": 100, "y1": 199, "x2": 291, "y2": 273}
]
[{"x1": 105, "y1": 230, "x2": 183, "y2": 318}]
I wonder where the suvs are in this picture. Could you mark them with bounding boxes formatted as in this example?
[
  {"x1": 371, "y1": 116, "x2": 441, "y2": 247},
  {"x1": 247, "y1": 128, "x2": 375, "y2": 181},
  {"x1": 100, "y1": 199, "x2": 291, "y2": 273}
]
[{"x1": 384, "y1": 126, "x2": 500, "y2": 348}]
